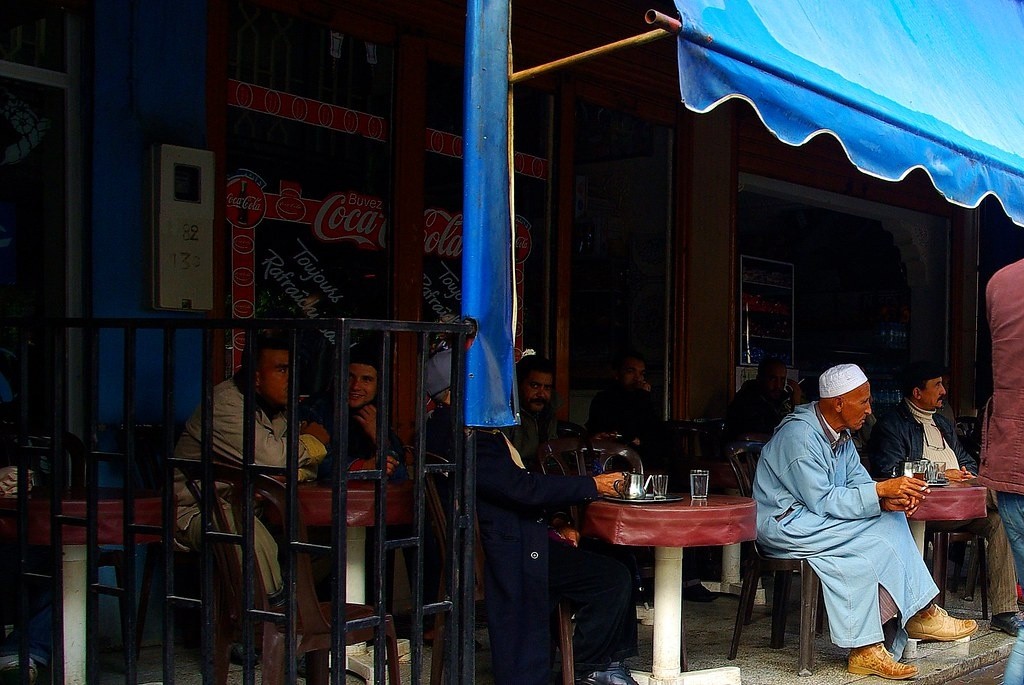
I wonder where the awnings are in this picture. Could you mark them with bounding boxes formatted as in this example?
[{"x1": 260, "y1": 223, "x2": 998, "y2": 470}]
[{"x1": 512, "y1": 1, "x2": 1024, "y2": 229}]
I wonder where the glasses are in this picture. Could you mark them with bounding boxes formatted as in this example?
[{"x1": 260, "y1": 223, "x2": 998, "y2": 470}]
[{"x1": 921, "y1": 423, "x2": 946, "y2": 451}]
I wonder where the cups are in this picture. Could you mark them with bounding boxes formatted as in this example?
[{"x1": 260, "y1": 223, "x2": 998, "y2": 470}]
[
  {"x1": 912, "y1": 459, "x2": 946, "y2": 483},
  {"x1": 652, "y1": 474, "x2": 668, "y2": 499},
  {"x1": 690, "y1": 469, "x2": 709, "y2": 500}
]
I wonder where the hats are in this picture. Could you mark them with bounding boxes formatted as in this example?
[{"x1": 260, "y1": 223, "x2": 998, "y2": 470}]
[
  {"x1": 818, "y1": 364, "x2": 868, "y2": 398},
  {"x1": 900, "y1": 358, "x2": 942, "y2": 382},
  {"x1": 428, "y1": 350, "x2": 452, "y2": 395}
]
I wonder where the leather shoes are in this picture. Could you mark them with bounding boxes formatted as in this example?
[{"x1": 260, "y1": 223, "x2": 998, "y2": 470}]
[{"x1": 584, "y1": 661, "x2": 639, "y2": 685}]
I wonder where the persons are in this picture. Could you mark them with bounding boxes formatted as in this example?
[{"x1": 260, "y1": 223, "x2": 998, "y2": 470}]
[
  {"x1": 587, "y1": 353, "x2": 719, "y2": 601},
  {"x1": 866, "y1": 359, "x2": 1024, "y2": 637},
  {"x1": 0, "y1": 370, "x2": 53, "y2": 685},
  {"x1": 977, "y1": 258, "x2": 1024, "y2": 685},
  {"x1": 729, "y1": 357, "x2": 802, "y2": 441},
  {"x1": 503, "y1": 356, "x2": 559, "y2": 468},
  {"x1": 296, "y1": 347, "x2": 410, "y2": 601},
  {"x1": 751, "y1": 363, "x2": 980, "y2": 679},
  {"x1": 172, "y1": 335, "x2": 329, "y2": 674},
  {"x1": 425, "y1": 351, "x2": 640, "y2": 685}
]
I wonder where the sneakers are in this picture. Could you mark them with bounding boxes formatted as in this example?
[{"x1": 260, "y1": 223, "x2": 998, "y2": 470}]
[
  {"x1": 905, "y1": 603, "x2": 978, "y2": 640},
  {"x1": 848, "y1": 643, "x2": 918, "y2": 680}
]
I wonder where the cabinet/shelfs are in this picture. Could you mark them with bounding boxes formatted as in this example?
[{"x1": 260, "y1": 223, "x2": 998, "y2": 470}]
[{"x1": 733, "y1": 278, "x2": 794, "y2": 371}]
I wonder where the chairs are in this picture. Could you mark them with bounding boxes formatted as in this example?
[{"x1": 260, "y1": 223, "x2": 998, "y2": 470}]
[{"x1": 125, "y1": 418, "x2": 989, "y2": 685}]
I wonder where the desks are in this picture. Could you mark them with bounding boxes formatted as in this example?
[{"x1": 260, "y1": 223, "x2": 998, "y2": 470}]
[
  {"x1": 583, "y1": 495, "x2": 757, "y2": 685},
  {"x1": 267, "y1": 479, "x2": 420, "y2": 685},
  {"x1": 11, "y1": 489, "x2": 178, "y2": 685},
  {"x1": 880, "y1": 481, "x2": 987, "y2": 609}
]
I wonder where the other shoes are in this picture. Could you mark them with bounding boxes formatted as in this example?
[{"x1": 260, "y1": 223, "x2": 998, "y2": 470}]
[
  {"x1": 991, "y1": 613, "x2": 1024, "y2": 637},
  {"x1": 683, "y1": 584, "x2": 719, "y2": 602},
  {"x1": 267, "y1": 585, "x2": 289, "y2": 607}
]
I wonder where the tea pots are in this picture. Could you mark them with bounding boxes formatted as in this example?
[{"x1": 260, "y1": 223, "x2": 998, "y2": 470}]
[
  {"x1": 892, "y1": 457, "x2": 915, "y2": 478},
  {"x1": 614, "y1": 467, "x2": 652, "y2": 500}
]
